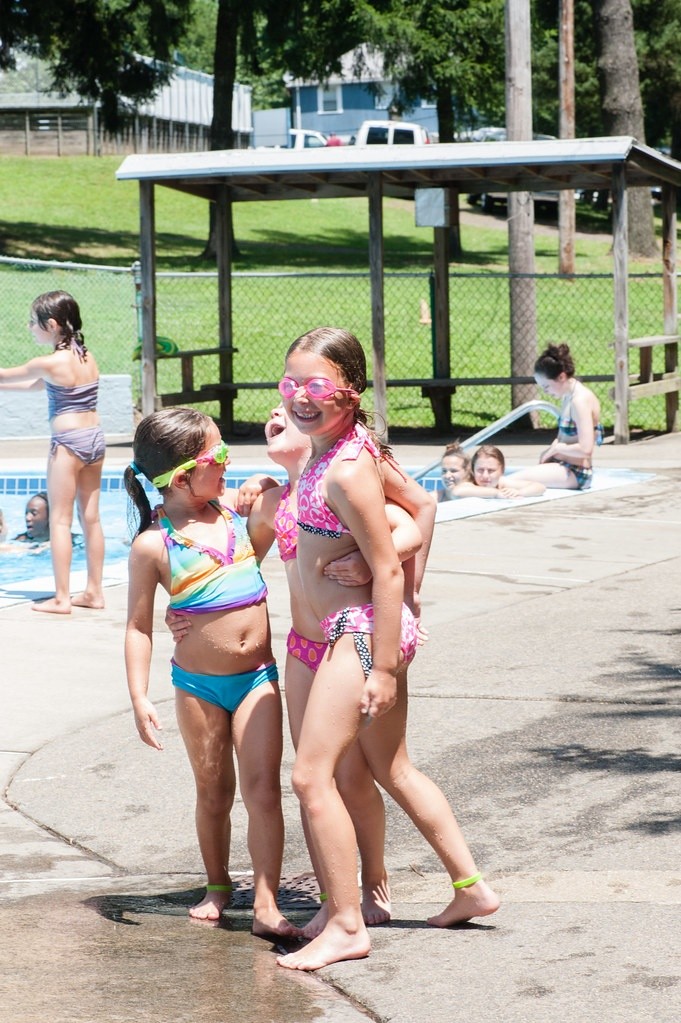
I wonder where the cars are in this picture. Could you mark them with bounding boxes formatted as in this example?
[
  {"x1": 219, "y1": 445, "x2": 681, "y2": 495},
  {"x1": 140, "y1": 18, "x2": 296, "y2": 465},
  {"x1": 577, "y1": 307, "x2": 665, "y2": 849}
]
[{"x1": 249, "y1": 119, "x2": 673, "y2": 216}]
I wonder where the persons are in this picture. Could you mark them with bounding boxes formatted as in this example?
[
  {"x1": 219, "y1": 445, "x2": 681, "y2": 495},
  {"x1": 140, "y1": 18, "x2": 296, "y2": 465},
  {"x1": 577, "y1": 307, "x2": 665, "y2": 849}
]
[
  {"x1": 324, "y1": 131, "x2": 342, "y2": 147},
  {"x1": 450, "y1": 445, "x2": 546, "y2": 500},
  {"x1": 501, "y1": 342, "x2": 601, "y2": 490},
  {"x1": 125, "y1": 409, "x2": 302, "y2": 940},
  {"x1": 0, "y1": 509, "x2": 45, "y2": 554},
  {"x1": 0, "y1": 291, "x2": 106, "y2": 614},
  {"x1": 425, "y1": 444, "x2": 471, "y2": 502},
  {"x1": 274, "y1": 327, "x2": 500, "y2": 971},
  {"x1": 14, "y1": 493, "x2": 51, "y2": 543},
  {"x1": 242, "y1": 400, "x2": 424, "y2": 940}
]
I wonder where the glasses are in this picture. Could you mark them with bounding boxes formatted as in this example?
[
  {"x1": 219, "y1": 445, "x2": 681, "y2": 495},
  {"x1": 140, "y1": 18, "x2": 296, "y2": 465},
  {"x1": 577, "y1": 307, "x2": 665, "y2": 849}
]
[
  {"x1": 277, "y1": 378, "x2": 356, "y2": 399},
  {"x1": 152, "y1": 440, "x2": 228, "y2": 487}
]
